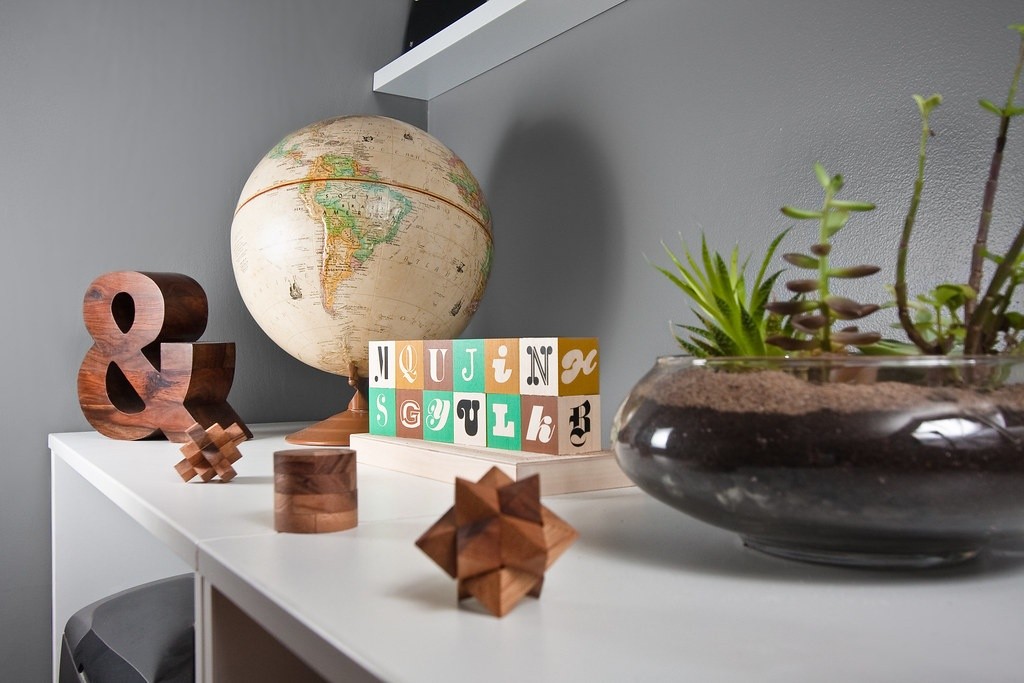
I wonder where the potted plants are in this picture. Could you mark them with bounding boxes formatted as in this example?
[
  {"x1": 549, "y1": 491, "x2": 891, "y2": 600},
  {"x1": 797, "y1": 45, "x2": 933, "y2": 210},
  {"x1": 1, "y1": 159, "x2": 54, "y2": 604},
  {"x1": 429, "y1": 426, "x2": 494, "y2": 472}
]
[{"x1": 608, "y1": 11, "x2": 1024, "y2": 568}]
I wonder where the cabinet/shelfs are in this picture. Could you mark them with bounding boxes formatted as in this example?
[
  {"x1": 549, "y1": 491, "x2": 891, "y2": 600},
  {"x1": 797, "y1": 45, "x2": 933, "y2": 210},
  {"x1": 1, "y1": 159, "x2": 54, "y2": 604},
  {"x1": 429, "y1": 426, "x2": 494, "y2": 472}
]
[{"x1": 37, "y1": 429, "x2": 1024, "y2": 680}]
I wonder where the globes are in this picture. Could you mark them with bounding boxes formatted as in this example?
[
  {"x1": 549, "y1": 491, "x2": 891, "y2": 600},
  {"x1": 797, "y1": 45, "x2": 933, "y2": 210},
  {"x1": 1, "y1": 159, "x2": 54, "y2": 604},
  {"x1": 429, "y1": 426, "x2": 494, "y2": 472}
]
[{"x1": 229, "y1": 115, "x2": 496, "y2": 446}]
